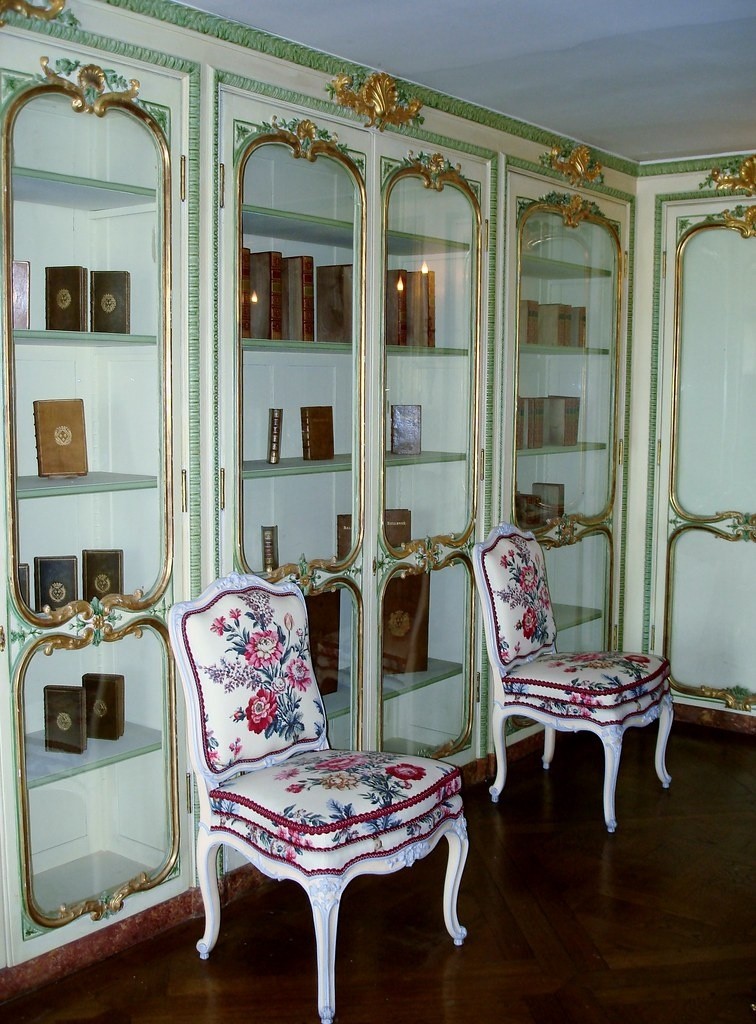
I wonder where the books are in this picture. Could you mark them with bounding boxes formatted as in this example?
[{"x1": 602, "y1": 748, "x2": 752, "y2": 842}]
[
  {"x1": 391, "y1": 403, "x2": 422, "y2": 456},
  {"x1": 46, "y1": 266, "x2": 86, "y2": 330},
  {"x1": 242, "y1": 248, "x2": 435, "y2": 348},
  {"x1": 11, "y1": 259, "x2": 30, "y2": 329},
  {"x1": 522, "y1": 296, "x2": 586, "y2": 527},
  {"x1": 301, "y1": 405, "x2": 335, "y2": 461},
  {"x1": 260, "y1": 523, "x2": 281, "y2": 572},
  {"x1": 18, "y1": 563, "x2": 30, "y2": 608},
  {"x1": 35, "y1": 556, "x2": 81, "y2": 614},
  {"x1": 83, "y1": 670, "x2": 125, "y2": 740},
  {"x1": 301, "y1": 588, "x2": 340, "y2": 699},
  {"x1": 382, "y1": 570, "x2": 430, "y2": 674},
  {"x1": 41, "y1": 685, "x2": 87, "y2": 754},
  {"x1": 90, "y1": 270, "x2": 131, "y2": 334},
  {"x1": 35, "y1": 398, "x2": 89, "y2": 477},
  {"x1": 337, "y1": 506, "x2": 412, "y2": 561},
  {"x1": 267, "y1": 408, "x2": 286, "y2": 464},
  {"x1": 83, "y1": 550, "x2": 125, "y2": 600}
]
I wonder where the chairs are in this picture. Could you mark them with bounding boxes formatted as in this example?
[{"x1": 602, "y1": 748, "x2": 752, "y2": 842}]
[
  {"x1": 162, "y1": 567, "x2": 472, "y2": 1024},
  {"x1": 469, "y1": 522, "x2": 675, "y2": 831}
]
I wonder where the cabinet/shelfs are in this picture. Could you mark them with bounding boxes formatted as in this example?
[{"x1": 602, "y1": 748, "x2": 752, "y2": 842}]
[{"x1": 0, "y1": 0, "x2": 640, "y2": 1003}]
